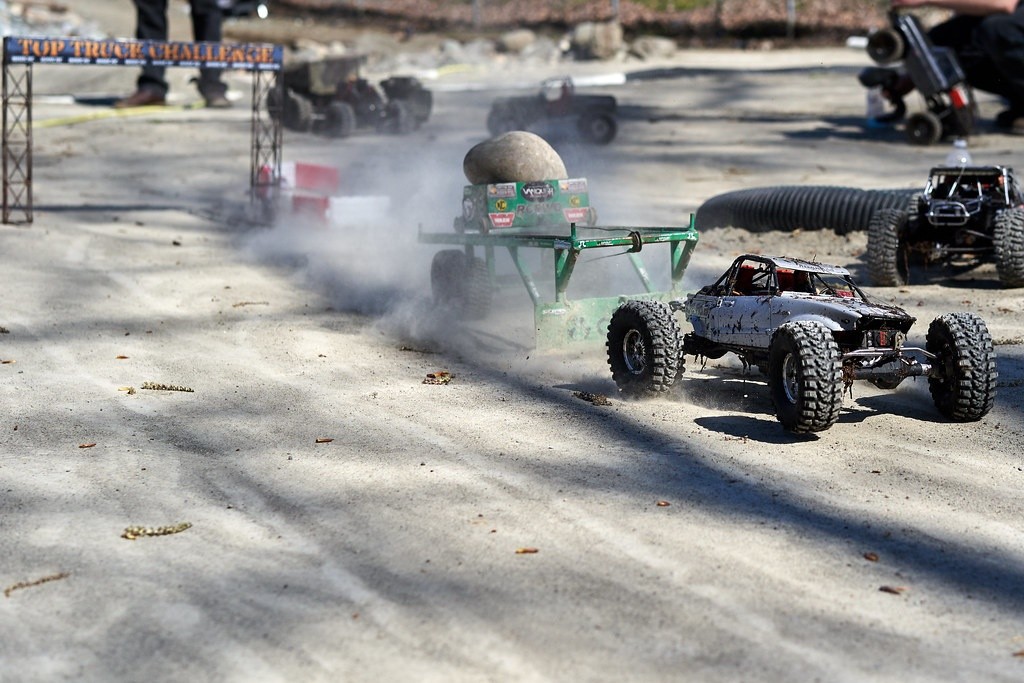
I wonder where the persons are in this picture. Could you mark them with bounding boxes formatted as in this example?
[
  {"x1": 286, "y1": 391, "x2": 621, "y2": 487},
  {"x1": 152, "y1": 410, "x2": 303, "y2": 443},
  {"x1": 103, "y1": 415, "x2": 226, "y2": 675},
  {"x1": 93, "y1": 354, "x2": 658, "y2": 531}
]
[
  {"x1": 114, "y1": 0, "x2": 232, "y2": 108},
  {"x1": 893, "y1": 0, "x2": 1024, "y2": 135}
]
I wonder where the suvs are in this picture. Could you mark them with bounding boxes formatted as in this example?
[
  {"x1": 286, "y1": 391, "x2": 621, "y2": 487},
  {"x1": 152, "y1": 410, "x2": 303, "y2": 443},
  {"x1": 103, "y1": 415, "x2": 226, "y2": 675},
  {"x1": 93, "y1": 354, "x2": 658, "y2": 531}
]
[
  {"x1": 602, "y1": 252, "x2": 998, "y2": 438},
  {"x1": 489, "y1": 78, "x2": 619, "y2": 150},
  {"x1": 854, "y1": 7, "x2": 978, "y2": 142},
  {"x1": 867, "y1": 160, "x2": 1024, "y2": 294},
  {"x1": 268, "y1": 51, "x2": 408, "y2": 140}
]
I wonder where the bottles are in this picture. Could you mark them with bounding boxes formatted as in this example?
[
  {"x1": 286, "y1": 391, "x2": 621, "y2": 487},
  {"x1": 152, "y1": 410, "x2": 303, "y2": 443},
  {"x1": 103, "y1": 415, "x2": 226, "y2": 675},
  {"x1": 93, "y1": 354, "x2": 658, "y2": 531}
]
[{"x1": 944, "y1": 137, "x2": 974, "y2": 168}]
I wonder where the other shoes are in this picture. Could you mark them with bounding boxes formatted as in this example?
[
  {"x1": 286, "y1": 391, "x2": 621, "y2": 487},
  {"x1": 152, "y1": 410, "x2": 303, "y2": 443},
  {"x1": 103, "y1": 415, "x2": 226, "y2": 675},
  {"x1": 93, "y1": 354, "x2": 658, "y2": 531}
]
[
  {"x1": 197, "y1": 80, "x2": 232, "y2": 107},
  {"x1": 115, "y1": 74, "x2": 169, "y2": 107}
]
